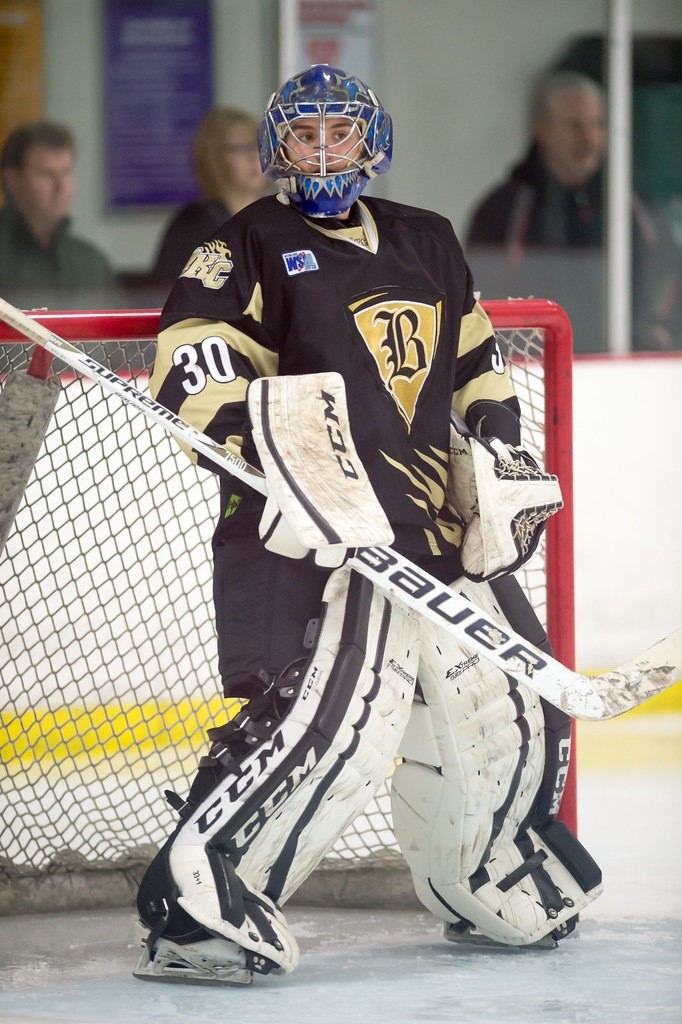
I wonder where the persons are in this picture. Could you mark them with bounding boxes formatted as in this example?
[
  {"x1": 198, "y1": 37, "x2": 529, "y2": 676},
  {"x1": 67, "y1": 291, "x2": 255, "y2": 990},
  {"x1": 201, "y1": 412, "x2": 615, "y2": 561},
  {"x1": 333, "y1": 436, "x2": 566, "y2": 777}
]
[
  {"x1": 147, "y1": 107, "x2": 268, "y2": 309},
  {"x1": 1, "y1": 120, "x2": 127, "y2": 318},
  {"x1": 461, "y1": 69, "x2": 680, "y2": 355},
  {"x1": 132, "y1": 61, "x2": 610, "y2": 989}
]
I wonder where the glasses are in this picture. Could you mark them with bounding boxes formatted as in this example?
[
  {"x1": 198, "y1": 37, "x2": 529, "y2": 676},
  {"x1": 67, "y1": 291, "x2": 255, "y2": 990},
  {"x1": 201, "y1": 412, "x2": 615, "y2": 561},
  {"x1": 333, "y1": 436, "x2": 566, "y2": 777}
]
[
  {"x1": 202, "y1": 137, "x2": 261, "y2": 161},
  {"x1": 537, "y1": 112, "x2": 607, "y2": 139}
]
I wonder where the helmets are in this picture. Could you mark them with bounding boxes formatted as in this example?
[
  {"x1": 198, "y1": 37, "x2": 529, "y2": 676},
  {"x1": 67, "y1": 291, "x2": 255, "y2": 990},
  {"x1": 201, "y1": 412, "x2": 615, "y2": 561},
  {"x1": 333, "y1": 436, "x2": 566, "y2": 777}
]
[{"x1": 256, "y1": 62, "x2": 394, "y2": 219}]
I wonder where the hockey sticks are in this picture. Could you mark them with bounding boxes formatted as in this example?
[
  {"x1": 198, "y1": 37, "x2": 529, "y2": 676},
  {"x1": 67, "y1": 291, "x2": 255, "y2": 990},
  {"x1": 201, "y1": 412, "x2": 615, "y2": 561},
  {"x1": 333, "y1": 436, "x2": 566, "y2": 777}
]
[{"x1": 0, "y1": 297, "x2": 682, "y2": 724}]
